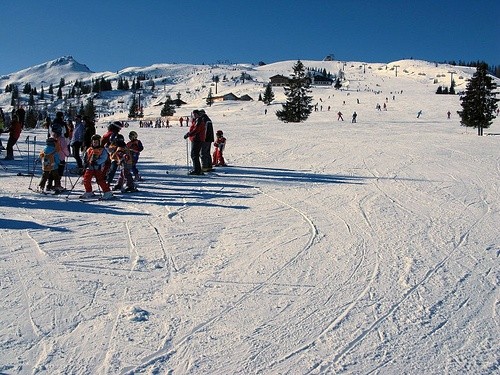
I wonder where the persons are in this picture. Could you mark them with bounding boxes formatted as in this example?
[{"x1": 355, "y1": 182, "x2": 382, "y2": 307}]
[
  {"x1": 184, "y1": 109, "x2": 214, "y2": 175},
  {"x1": 179, "y1": 117, "x2": 183, "y2": 127},
  {"x1": 212, "y1": 131, "x2": 227, "y2": 167},
  {"x1": 314, "y1": 89, "x2": 404, "y2": 123},
  {"x1": 0, "y1": 107, "x2": 21, "y2": 160},
  {"x1": 447, "y1": 111, "x2": 451, "y2": 118},
  {"x1": 37, "y1": 112, "x2": 144, "y2": 201},
  {"x1": 15, "y1": 105, "x2": 26, "y2": 131},
  {"x1": 417, "y1": 110, "x2": 422, "y2": 118},
  {"x1": 139, "y1": 119, "x2": 170, "y2": 128}
]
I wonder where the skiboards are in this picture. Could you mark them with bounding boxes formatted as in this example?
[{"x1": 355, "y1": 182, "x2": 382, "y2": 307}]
[
  {"x1": 166, "y1": 164, "x2": 233, "y2": 177},
  {"x1": 65, "y1": 195, "x2": 105, "y2": 200},
  {"x1": 80, "y1": 195, "x2": 125, "y2": 202}
]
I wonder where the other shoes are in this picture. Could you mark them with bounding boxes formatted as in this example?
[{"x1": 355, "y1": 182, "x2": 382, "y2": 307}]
[
  {"x1": 124, "y1": 185, "x2": 135, "y2": 192},
  {"x1": 55, "y1": 186, "x2": 65, "y2": 191},
  {"x1": 99, "y1": 191, "x2": 114, "y2": 200},
  {"x1": 79, "y1": 191, "x2": 95, "y2": 199},
  {"x1": 114, "y1": 183, "x2": 123, "y2": 188}
]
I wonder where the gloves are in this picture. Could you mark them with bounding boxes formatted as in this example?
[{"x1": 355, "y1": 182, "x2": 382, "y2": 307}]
[{"x1": 184, "y1": 133, "x2": 189, "y2": 139}]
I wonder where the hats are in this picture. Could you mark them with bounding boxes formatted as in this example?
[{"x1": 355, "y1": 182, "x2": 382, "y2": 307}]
[
  {"x1": 114, "y1": 134, "x2": 124, "y2": 143},
  {"x1": 129, "y1": 131, "x2": 138, "y2": 140},
  {"x1": 49, "y1": 122, "x2": 62, "y2": 138},
  {"x1": 109, "y1": 133, "x2": 116, "y2": 143},
  {"x1": 46, "y1": 138, "x2": 58, "y2": 147}
]
